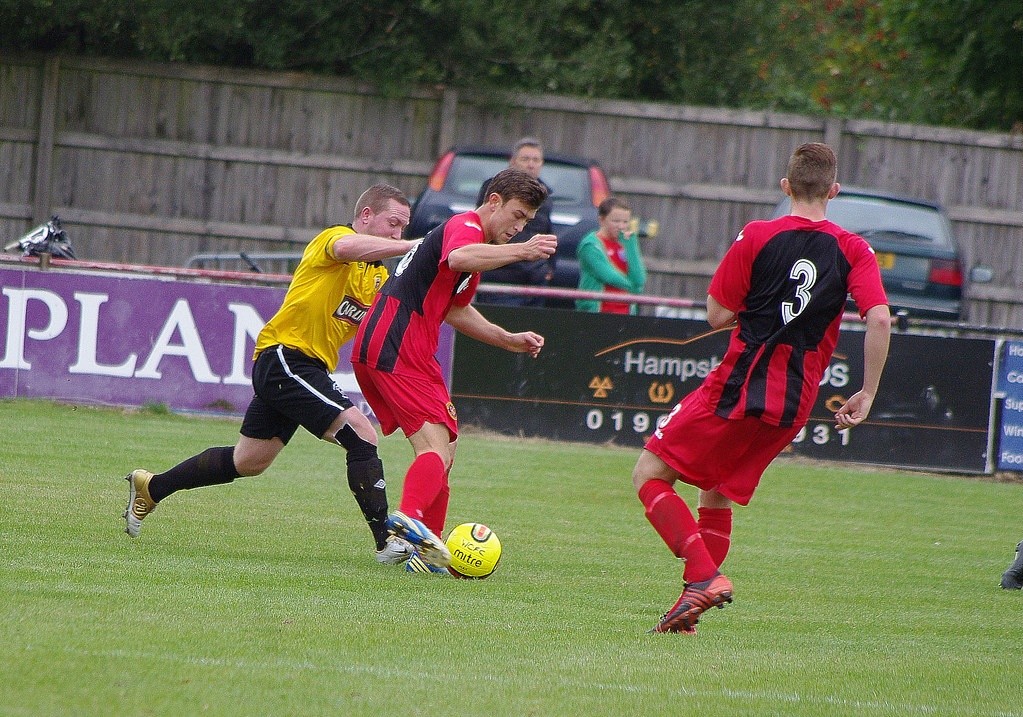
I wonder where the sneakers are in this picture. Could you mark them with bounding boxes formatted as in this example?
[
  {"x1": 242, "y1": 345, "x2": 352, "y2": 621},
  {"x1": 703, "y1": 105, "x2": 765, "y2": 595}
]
[
  {"x1": 406, "y1": 550, "x2": 453, "y2": 577},
  {"x1": 375, "y1": 534, "x2": 415, "y2": 565},
  {"x1": 649, "y1": 574, "x2": 734, "y2": 635},
  {"x1": 123, "y1": 469, "x2": 158, "y2": 539},
  {"x1": 386, "y1": 509, "x2": 451, "y2": 568}
]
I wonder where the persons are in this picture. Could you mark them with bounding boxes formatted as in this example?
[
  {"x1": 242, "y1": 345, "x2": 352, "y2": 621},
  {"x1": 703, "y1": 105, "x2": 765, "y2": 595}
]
[
  {"x1": 478, "y1": 136, "x2": 556, "y2": 304},
  {"x1": 123, "y1": 183, "x2": 425, "y2": 566},
  {"x1": 574, "y1": 197, "x2": 648, "y2": 314},
  {"x1": 351, "y1": 169, "x2": 558, "y2": 577},
  {"x1": 632, "y1": 142, "x2": 892, "y2": 632}
]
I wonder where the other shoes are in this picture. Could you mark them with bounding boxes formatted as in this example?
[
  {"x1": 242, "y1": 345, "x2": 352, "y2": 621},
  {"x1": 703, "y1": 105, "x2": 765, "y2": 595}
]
[{"x1": 999, "y1": 540, "x2": 1023, "y2": 590}]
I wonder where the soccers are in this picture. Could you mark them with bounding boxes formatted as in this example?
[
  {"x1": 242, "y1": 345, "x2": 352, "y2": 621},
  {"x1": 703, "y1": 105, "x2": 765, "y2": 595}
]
[{"x1": 442, "y1": 522, "x2": 504, "y2": 581}]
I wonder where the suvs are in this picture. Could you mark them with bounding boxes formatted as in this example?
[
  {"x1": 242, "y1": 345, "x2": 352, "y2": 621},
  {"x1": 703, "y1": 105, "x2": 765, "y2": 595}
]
[
  {"x1": 773, "y1": 184, "x2": 966, "y2": 327},
  {"x1": 401, "y1": 143, "x2": 660, "y2": 307}
]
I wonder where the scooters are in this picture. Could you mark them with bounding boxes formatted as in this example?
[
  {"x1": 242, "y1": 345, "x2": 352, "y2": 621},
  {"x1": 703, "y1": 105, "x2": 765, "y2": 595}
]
[{"x1": 3, "y1": 214, "x2": 78, "y2": 259}]
